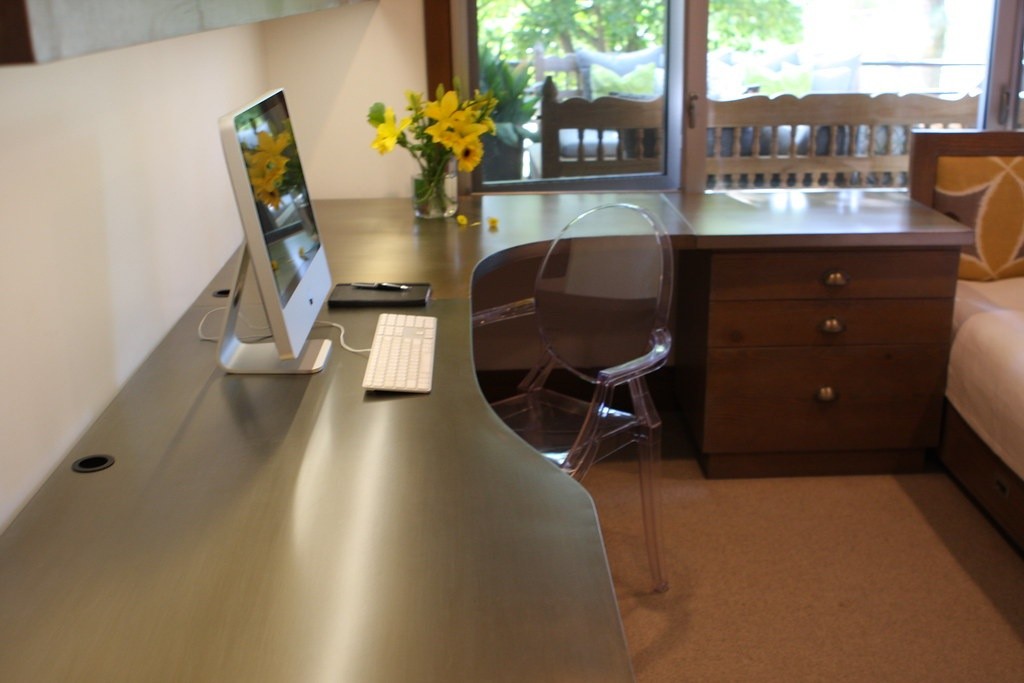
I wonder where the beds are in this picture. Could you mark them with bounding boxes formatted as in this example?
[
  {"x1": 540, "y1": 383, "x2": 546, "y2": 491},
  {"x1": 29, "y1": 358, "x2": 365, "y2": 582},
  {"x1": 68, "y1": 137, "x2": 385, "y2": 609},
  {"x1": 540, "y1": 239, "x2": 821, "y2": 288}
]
[{"x1": 909, "y1": 275, "x2": 1024, "y2": 552}]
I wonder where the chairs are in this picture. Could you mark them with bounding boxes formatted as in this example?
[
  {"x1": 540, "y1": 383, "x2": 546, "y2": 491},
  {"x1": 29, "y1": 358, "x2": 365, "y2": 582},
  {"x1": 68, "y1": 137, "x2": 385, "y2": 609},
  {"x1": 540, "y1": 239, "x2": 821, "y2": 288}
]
[{"x1": 472, "y1": 203, "x2": 674, "y2": 593}]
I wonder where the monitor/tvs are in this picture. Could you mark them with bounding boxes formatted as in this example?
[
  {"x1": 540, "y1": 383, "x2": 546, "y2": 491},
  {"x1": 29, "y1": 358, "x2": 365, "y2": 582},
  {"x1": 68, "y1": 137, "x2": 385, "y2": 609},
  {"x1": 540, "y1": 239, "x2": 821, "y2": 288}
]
[{"x1": 218, "y1": 87, "x2": 333, "y2": 361}]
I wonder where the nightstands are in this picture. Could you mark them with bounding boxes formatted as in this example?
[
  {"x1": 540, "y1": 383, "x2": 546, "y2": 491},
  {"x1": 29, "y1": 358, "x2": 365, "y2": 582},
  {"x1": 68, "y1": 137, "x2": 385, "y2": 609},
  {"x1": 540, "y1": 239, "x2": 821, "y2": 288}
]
[{"x1": 661, "y1": 192, "x2": 976, "y2": 481}]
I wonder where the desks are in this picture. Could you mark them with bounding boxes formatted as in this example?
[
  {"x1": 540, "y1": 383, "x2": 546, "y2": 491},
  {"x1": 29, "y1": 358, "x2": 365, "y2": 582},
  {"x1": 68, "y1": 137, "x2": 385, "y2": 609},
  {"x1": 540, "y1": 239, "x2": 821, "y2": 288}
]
[{"x1": 0, "y1": 197, "x2": 634, "y2": 683}]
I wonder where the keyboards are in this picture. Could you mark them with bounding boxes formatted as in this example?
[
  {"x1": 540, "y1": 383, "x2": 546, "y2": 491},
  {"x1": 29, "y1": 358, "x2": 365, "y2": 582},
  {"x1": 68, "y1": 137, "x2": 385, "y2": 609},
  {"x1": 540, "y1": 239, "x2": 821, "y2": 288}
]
[{"x1": 361, "y1": 313, "x2": 437, "y2": 395}]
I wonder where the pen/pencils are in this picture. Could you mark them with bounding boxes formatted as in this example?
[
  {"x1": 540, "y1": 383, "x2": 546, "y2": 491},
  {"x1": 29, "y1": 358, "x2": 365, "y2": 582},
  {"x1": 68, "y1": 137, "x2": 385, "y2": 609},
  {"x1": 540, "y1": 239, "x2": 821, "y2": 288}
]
[{"x1": 350, "y1": 284, "x2": 410, "y2": 291}]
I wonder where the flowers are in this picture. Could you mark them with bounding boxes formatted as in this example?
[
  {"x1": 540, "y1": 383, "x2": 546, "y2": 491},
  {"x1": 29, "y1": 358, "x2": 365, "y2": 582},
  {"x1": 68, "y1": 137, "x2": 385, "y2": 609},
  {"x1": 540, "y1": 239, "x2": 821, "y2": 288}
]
[{"x1": 367, "y1": 75, "x2": 499, "y2": 172}]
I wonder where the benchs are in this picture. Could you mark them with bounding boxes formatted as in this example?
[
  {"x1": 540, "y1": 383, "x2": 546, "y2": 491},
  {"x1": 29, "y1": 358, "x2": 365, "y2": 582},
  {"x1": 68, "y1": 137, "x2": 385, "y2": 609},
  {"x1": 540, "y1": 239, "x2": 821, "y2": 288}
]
[{"x1": 542, "y1": 76, "x2": 980, "y2": 188}]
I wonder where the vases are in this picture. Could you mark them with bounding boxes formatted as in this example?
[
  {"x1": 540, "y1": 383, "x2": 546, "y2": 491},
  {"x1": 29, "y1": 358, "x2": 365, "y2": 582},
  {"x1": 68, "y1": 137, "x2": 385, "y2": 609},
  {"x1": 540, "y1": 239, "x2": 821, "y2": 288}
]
[{"x1": 410, "y1": 157, "x2": 457, "y2": 219}]
